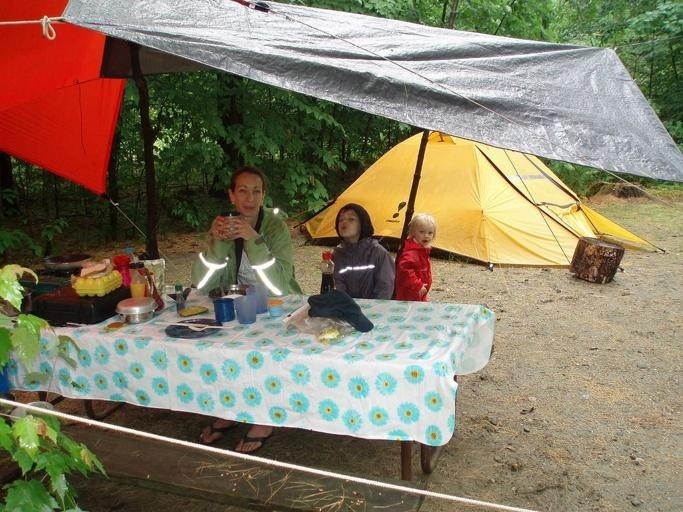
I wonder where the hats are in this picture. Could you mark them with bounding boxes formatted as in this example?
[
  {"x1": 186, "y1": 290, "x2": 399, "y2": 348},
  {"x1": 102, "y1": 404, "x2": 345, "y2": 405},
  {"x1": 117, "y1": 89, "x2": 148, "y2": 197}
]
[{"x1": 307, "y1": 289, "x2": 374, "y2": 332}]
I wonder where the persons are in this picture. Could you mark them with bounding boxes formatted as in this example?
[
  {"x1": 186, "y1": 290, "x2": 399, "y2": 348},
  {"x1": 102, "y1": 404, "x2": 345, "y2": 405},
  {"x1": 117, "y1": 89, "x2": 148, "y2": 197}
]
[
  {"x1": 191, "y1": 166, "x2": 306, "y2": 296},
  {"x1": 393, "y1": 211, "x2": 438, "y2": 303},
  {"x1": 331, "y1": 202, "x2": 397, "y2": 300}
]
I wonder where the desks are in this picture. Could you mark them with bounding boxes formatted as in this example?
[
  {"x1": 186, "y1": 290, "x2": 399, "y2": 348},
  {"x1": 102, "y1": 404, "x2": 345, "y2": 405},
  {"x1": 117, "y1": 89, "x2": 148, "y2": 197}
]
[{"x1": 0, "y1": 287, "x2": 495, "y2": 479}]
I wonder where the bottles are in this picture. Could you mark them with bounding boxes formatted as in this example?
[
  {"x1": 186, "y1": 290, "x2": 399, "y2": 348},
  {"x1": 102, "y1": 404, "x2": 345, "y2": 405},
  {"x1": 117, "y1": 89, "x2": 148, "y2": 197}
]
[
  {"x1": 319, "y1": 252, "x2": 337, "y2": 298},
  {"x1": 176, "y1": 284, "x2": 186, "y2": 316},
  {"x1": 130, "y1": 263, "x2": 146, "y2": 299}
]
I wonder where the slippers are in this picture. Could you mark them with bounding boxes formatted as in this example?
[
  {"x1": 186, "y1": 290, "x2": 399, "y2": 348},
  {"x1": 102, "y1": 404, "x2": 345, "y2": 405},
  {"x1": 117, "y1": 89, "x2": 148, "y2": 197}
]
[
  {"x1": 198, "y1": 424, "x2": 240, "y2": 445},
  {"x1": 233, "y1": 431, "x2": 275, "y2": 453}
]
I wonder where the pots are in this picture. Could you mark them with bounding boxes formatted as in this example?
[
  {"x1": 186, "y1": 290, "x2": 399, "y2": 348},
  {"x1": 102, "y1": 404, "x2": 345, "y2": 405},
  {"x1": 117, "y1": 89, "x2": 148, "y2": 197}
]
[
  {"x1": 115, "y1": 298, "x2": 158, "y2": 324},
  {"x1": 39, "y1": 252, "x2": 97, "y2": 271}
]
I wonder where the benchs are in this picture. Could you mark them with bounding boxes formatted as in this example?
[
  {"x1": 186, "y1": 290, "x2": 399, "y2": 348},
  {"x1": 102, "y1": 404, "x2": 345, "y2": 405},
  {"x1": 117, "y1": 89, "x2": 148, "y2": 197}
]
[{"x1": 0, "y1": 423, "x2": 426, "y2": 512}]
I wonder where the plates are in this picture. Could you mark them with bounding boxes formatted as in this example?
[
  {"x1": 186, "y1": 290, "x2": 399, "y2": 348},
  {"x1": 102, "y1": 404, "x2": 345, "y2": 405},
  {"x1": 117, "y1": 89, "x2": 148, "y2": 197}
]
[{"x1": 165, "y1": 318, "x2": 223, "y2": 339}]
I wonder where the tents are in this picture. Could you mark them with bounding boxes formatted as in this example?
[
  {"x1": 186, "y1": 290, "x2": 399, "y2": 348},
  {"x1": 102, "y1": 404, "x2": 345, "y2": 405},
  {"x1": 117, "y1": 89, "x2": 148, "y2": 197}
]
[{"x1": 302, "y1": 128, "x2": 669, "y2": 274}]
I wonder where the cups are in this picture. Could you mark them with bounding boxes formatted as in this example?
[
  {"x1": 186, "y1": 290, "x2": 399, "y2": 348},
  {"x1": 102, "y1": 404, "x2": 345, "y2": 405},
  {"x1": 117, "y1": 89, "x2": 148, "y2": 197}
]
[
  {"x1": 245, "y1": 287, "x2": 267, "y2": 313},
  {"x1": 130, "y1": 279, "x2": 144, "y2": 298},
  {"x1": 232, "y1": 296, "x2": 257, "y2": 323},
  {"x1": 213, "y1": 298, "x2": 236, "y2": 323}
]
